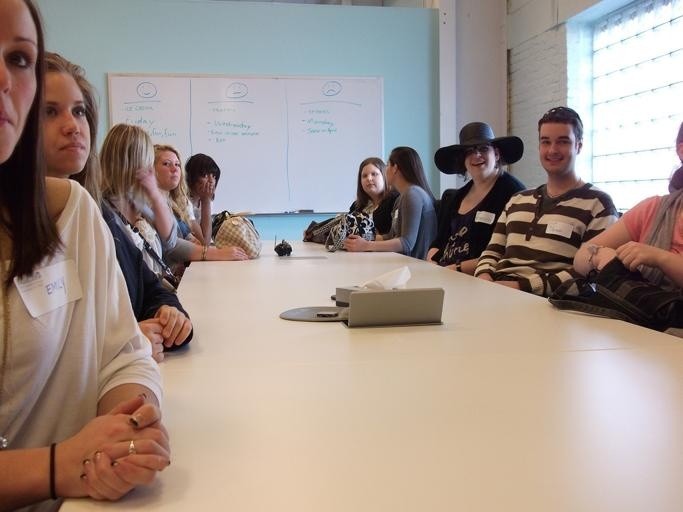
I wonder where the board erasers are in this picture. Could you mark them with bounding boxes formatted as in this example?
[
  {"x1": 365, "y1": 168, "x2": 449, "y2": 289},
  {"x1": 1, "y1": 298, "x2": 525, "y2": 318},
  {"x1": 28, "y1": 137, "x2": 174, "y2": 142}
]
[{"x1": 299, "y1": 210, "x2": 313, "y2": 213}]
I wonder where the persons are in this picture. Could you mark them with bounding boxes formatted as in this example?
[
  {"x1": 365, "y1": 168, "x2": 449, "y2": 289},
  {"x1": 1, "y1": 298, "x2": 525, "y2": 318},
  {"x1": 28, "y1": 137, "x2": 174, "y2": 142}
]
[
  {"x1": 425, "y1": 122, "x2": 527, "y2": 272},
  {"x1": 97, "y1": 124, "x2": 179, "y2": 293},
  {"x1": 342, "y1": 147, "x2": 438, "y2": 260},
  {"x1": 40, "y1": 49, "x2": 192, "y2": 361},
  {"x1": 0, "y1": 0, "x2": 171, "y2": 511},
  {"x1": 474, "y1": 107, "x2": 618, "y2": 296},
  {"x1": 349, "y1": 158, "x2": 399, "y2": 232},
  {"x1": 153, "y1": 143, "x2": 250, "y2": 277},
  {"x1": 185, "y1": 154, "x2": 220, "y2": 246}
]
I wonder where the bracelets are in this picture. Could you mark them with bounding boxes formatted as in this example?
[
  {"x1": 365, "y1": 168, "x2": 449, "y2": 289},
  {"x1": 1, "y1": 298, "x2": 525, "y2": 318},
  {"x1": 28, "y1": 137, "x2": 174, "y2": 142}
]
[
  {"x1": 454, "y1": 259, "x2": 461, "y2": 273},
  {"x1": 572, "y1": 123, "x2": 682, "y2": 288},
  {"x1": 47, "y1": 441, "x2": 62, "y2": 501},
  {"x1": 585, "y1": 244, "x2": 605, "y2": 263},
  {"x1": 200, "y1": 246, "x2": 207, "y2": 260}
]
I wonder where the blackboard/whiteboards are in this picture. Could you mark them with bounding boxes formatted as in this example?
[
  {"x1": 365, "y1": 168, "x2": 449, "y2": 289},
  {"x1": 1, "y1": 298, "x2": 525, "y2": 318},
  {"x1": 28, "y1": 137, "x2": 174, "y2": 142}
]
[{"x1": 107, "y1": 72, "x2": 384, "y2": 217}]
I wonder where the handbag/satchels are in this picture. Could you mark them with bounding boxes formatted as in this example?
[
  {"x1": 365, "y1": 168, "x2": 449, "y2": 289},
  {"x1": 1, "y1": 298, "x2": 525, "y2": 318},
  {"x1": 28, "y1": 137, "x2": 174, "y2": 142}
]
[
  {"x1": 214, "y1": 216, "x2": 262, "y2": 258},
  {"x1": 303, "y1": 218, "x2": 335, "y2": 244},
  {"x1": 548, "y1": 258, "x2": 683, "y2": 330},
  {"x1": 326, "y1": 210, "x2": 377, "y2": 252},
  {"x1": 213, "y1": 211, "x2": 259, "y2": 243}
]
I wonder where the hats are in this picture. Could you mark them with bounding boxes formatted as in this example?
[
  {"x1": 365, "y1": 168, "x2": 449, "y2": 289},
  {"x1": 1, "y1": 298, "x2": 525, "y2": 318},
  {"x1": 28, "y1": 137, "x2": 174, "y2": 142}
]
[{"x1": 434, "y1": 122, "x2": 524, "y2": 174}]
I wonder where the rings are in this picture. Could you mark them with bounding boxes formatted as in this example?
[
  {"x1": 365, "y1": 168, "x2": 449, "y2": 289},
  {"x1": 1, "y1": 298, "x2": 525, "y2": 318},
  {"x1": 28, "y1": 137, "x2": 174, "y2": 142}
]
[{"x1": 125, "y1": 439, "x2": 139, "y2": 457}]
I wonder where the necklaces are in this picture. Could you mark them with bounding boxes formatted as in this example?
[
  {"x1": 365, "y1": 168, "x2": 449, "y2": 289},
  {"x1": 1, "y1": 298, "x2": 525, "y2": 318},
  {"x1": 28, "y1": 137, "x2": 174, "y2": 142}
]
[{"x1": 548, "y1": 176, "x2": 581, "y2": 198}]
